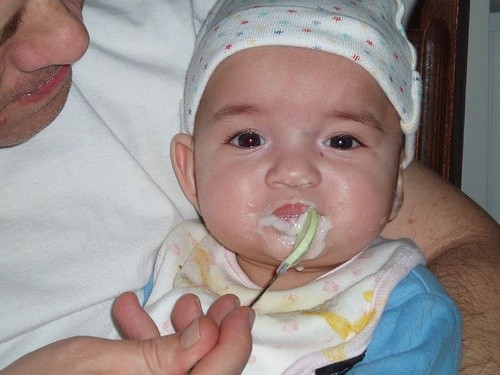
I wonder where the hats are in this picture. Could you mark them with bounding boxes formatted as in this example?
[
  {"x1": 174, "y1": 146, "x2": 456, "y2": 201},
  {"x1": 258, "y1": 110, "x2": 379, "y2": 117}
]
[{"x1": 183, "y1": 0, "x2": 422, "y2": 169}]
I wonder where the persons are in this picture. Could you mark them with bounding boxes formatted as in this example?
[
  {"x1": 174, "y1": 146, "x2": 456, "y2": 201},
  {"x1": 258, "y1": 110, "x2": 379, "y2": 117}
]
[
  {"x1": 141, "y1": 0, "x2": 463, "y2": 375},
  {"x1": 0, "y1": 0, "x2": 500, "y2": 375}
]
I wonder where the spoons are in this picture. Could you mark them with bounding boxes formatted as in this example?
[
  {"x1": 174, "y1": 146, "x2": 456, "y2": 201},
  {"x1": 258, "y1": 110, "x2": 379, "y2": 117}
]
[{"x1": 248, "y1": 208, "x2": 318, "y2": 308}]
